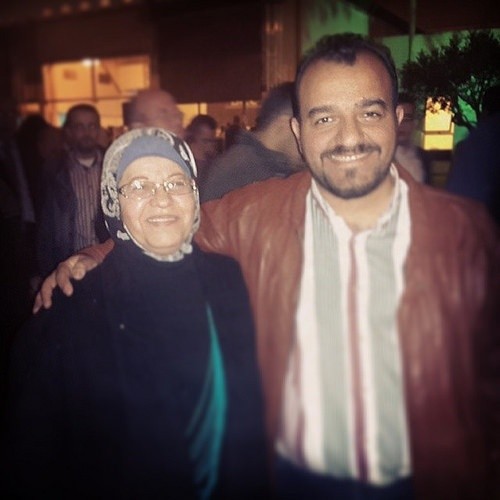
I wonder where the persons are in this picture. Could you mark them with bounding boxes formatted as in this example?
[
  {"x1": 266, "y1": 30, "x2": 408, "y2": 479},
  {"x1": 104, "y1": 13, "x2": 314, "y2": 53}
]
[
  {"x1": 35, "y1": 30, "x2": 500, "y2": 500},
  {"x1": 0, "y1": 79, "x2": 500, "y2": 500}
]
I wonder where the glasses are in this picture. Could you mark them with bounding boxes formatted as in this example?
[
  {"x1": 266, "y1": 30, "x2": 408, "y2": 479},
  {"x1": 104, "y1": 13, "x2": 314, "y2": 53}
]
[{"x1": 116, "y1": 175, "x2": 196, "y2": 199}]
